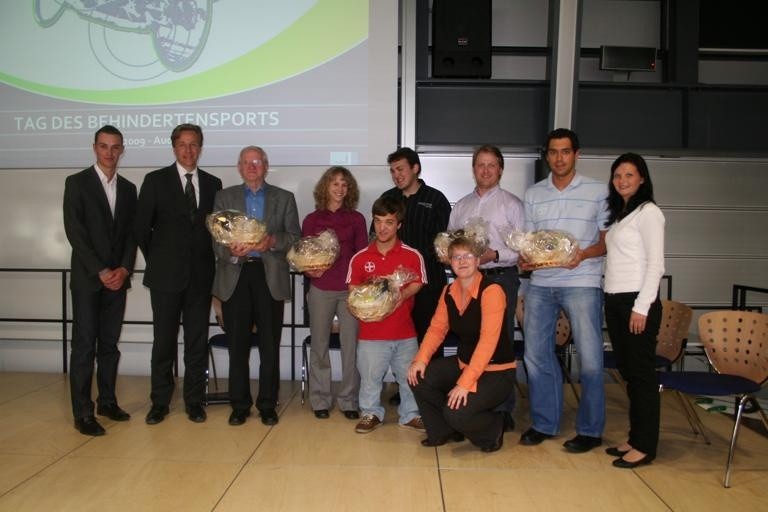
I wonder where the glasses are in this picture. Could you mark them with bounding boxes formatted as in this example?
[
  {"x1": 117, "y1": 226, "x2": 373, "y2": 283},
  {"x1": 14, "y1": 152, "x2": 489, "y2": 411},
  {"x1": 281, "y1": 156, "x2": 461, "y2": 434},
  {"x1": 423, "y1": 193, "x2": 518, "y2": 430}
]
[{"x1": 238, "y1": 159, "x2": 263, "y2": 166}]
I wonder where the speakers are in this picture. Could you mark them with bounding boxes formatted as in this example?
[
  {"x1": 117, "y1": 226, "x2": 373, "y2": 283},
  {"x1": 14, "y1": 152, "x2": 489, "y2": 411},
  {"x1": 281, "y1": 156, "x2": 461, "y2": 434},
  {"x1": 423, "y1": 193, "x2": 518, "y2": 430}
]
[
  {"x1": 599, "y1": 44, "x2": 659, "y2": 72},
  {"x1": 430, "y1": 0, "x2": 494, "y2": 79}
]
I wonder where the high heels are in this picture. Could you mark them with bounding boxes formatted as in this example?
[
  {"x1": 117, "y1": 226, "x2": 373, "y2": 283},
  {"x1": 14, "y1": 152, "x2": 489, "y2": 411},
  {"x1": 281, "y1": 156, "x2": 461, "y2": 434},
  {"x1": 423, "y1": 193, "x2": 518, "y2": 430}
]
[
  {"x1": 421, "y1": 431, "x2": 464, "y2": 446},
  {"x1": 481, "y1": 409, "x2": 514, "y2": 452}
]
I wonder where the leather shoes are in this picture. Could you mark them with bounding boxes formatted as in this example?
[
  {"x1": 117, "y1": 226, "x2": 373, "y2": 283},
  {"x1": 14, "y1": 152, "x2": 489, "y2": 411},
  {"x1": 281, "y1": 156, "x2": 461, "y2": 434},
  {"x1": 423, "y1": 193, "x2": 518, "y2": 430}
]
[
  {"x1": 344, "y1": 410, "x2": 359, "y2": 419},
  {"x1": 563, "y1": 434, "x2": 602, "y2": 452},
  {"x1": 613, "y1": 449, "x2": 657, "y2": 468},
  {"x1": 315, "y1": 409, "x2": 328, "y2": 418},
  {"x1": 146, "y1": 403, "x2": 169, "y2": 424},
  {"x1": 74, "y1": 417, "x2": 105, "y2": 435},
  {"x1": 260, "y1": 409, "x2": 278, "y2": 425},
  {"x1": 605, "y1": 443, "x2": 634, "y2": 456},
  {"x1": 389, "y1": 391, "x2": 400, "y2": 404},
  {"x1": 185, "y1": 405, "x2": 206, "y2": 423},
  {"x1": 229, "y1": 405, "x2": 251, "y2": 425},
  {"x1": 97, "y1": 404, "x2": 130, "y2": 420},
  {"x1": 519, "y1": 428, "x2": 559, "y2": 444}
]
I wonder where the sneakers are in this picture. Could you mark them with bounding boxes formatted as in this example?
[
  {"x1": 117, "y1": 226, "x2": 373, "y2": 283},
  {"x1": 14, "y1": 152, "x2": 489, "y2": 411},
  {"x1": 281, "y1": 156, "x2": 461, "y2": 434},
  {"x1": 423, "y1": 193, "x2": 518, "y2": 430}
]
[
  {"x1": 400, "y1": 416, "x2": 427, "y2": 433},
  {"x1": 356, "y1": 413, "x2": 382, "y2": 433}
]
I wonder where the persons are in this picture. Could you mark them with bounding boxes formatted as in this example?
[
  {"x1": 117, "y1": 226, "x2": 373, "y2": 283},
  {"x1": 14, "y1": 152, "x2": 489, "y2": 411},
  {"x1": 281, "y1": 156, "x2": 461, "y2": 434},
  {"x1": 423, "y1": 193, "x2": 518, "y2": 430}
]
[
  {"x1": 211, "y1": 146, "x2": 301, "y2": 427},
  {"x1": 446, "y1": 146, "x2": 526, "y2": 432},
  {"x1": 302, "y1": 166, "x2": 368, "y2": 420},
  {"x1": 605, "y1": 152, "x2": 665, "y2": 467},
  {"x1": 367, "y1": 147, "x2": 452, "y2": 404},
  {"x1": 406, "y1": 238, "x2": 517, "y2": 452},
  {"x1": 62, "y1": 125, "x2": 137, "y2": 435},
  {"x1": 519, "y1": 129, "x2": 609, "y2": 452},
  {"x1": 345, "y1": 195, "x2": 427, "y2": 434},
  {"x1": 137, "y1": 123, "x2": 223, "y2": 425}
]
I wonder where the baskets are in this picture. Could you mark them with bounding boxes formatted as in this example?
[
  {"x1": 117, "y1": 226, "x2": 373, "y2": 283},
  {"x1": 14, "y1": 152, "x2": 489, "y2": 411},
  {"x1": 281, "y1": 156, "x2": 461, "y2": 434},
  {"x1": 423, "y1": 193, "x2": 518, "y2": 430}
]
[
  {"x1": 289, "y1": 253, "x2": 334, "y2": 271},
  {"x1": 216, "y1": 230, "x2": 262, "y2": 244},
  {"x1": 347, "y1": 286, "x2": 392, "y2": 323},
  {"x1": 433, "y1": 237, "x2": 451, "y2": 262},
  {"x1": 522, "y1": 249, "x2": 567, "y2": 263}
]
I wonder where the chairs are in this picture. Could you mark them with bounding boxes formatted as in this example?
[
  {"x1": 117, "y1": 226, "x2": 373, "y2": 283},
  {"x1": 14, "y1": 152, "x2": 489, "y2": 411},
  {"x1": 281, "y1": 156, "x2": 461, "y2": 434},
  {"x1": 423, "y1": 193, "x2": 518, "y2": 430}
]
[
  {"x1": 300, "y1": 292, "x2": 340, "y2": 406},
  {"x1": 204, "y1": 316, "x2": 260, "y2": 409},
  {"x1": 658, "y1": 310, "x2": 767, "y2": 487},
  {"x1": 511, "y1": 294, "x2": 579, "y2": 406},
  {"x1": 602, "y1": 300, "x2": 711, "y2": 444}
]
